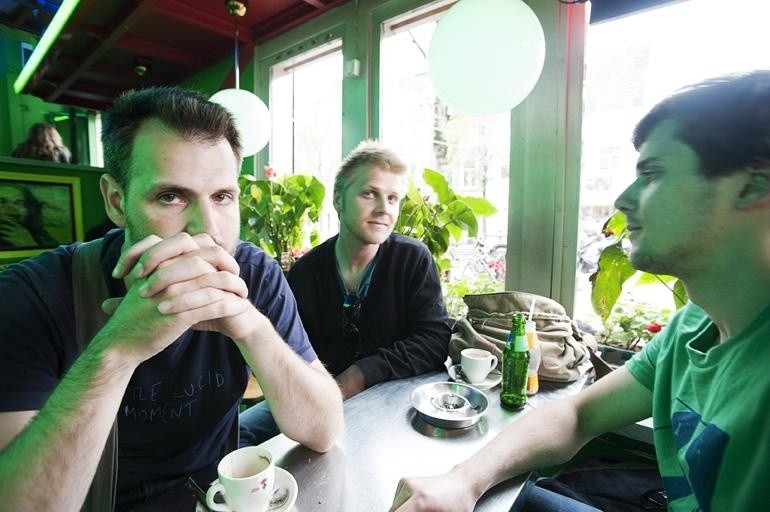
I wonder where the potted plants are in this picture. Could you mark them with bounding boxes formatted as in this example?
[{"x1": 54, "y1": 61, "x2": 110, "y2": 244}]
[{"x1": 238, "y1": 172, "x2": 327, "y2": 272}]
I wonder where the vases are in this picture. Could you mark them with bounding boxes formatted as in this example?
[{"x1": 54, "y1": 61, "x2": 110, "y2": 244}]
[{"x1": 595, "y1": 342, "x2": 642, "y2": 365}]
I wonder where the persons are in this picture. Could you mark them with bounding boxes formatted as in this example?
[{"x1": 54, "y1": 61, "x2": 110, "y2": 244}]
[
  {"x1": 388, "y1": 65, "x2": 770, "y2": 512},
  {"x1": 0, "y1": 182, "x2": 60, "y2": 249},
  {"x1": 1, "y1": 85, "x2": 345, "y2": 512},
  {"x1": 12, "y1": 116, "x2": 75, "y2": 164},
  {"x1": 237, "y1": 136, "x2": 452, "y2": 449}
]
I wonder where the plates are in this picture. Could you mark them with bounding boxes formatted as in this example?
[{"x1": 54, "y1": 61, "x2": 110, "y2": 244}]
[
  {"x1": 446, "y1": 363, "x2": 502, "y2": 391},
  {"x1": 410, "y1": 381, "x2": 489, "y2": 429},
  {"x1": 198, "y1": 466, "x2": 300, "y2": 512}
]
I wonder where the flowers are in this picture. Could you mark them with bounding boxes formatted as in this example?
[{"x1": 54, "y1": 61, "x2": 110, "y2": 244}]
[{"x1": 599, "y1": 304, "x2": 675, "y2": 351}]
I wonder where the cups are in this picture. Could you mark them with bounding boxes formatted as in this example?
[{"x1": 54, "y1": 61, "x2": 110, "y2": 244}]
[
  {"x1": 460, "y1": 347, "x2": 498, "y2": 381},
  {"x1": 206, "y1": 446, "x2": 276, "y2": 511}
]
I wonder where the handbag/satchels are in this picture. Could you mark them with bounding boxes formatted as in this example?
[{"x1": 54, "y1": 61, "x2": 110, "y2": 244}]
[{"x1": 449, "y1": 292, "x2": 598, "y2": 383}]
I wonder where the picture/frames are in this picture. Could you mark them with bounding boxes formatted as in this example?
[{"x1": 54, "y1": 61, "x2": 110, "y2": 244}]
[{"x1": 0, "y1": 171, "x2": 86, "y2": 259}]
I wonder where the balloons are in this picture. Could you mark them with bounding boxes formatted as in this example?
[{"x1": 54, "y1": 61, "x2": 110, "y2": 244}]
[{"x1": 208, "y1": 89, "x2": 272, "y2": 159}]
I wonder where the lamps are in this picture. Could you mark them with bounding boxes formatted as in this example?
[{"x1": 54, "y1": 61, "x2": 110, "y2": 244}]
[{"x1": 208, "y1": 0, "x2": 273, "y2": 157}]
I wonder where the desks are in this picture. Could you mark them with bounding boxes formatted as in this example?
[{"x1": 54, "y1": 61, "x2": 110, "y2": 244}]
[{"x1": 255, "y1": 369, "x2": 654, "y2": 512}]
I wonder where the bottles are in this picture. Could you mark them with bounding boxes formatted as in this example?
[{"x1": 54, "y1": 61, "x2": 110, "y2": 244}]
[
  {"x1": 523, "y1": 320, "x2": 541, "y2": 395},
  {"x1": 501, "y1": 313, "x2": 530, "y2": 410}
]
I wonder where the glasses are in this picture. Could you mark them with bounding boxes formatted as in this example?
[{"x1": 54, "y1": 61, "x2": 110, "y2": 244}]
[{"x1": 341, "y1": 294, "x2": 362, "y2": 340}]
